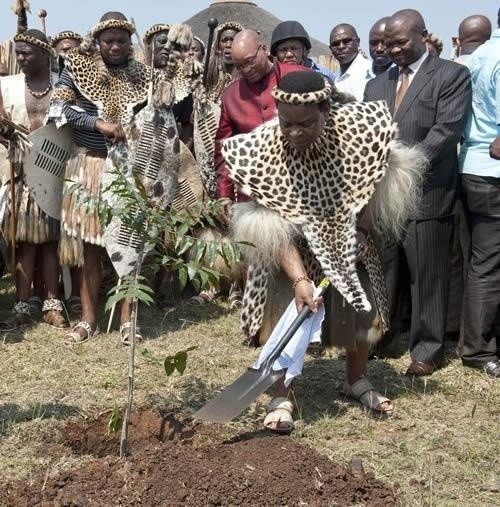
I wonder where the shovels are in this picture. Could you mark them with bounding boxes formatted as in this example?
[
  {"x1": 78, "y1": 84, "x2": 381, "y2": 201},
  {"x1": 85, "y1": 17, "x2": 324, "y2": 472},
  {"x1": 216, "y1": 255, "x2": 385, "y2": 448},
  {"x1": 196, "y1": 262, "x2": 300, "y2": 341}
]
[{"x1": 192, "y1": 243, "x2": 364, "y2": 422}]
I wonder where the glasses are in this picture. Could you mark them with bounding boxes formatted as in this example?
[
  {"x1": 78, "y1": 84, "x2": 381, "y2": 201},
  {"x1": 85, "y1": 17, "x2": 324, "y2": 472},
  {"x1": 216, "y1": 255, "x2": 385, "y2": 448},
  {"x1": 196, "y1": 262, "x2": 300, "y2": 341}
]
[{"x1": 236, "y1": 45, "x2": 261, "y2": 71}]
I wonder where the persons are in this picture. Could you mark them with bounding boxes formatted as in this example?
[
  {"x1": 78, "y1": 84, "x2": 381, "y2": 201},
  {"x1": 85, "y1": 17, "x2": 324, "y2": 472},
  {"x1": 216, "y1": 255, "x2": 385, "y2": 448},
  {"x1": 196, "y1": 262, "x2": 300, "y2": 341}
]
[
  {"x1": 329, "y1": 22, "x2": 375, "y2": 101},
  {"x1": 214, "y1": 28, "x2": 314, "y2": 344},
  {"x1": 451, "y1": 15, "x2": 491, "y2": 65},
  {"x1": 270, "y1": 20, "x2": 337, "y2": 82},
  {"x1": 0, "y1": 0, "x2": 244, "y2": 344},
  {"x1": 361, "y1": 16, "x2": 391, "y2": 81},
  {"x1": 219, "y1": 70, "x2": 393, "y2": 432},
  {"x1": 424, "y1": 33, "x2": 444, "y2": 58},
  {"x1": 362, "y1": 8, "x2": 472, "y2": 376},
  {"x1": 456, "y1": 8, "x2": 500, "y2": 379}
]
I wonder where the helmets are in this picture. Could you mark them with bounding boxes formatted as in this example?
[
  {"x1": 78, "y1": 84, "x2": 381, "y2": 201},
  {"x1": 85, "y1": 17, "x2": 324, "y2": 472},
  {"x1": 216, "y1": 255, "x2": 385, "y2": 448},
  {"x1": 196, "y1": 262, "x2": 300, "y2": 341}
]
[{"x1": 270, "y1": 21, "x2": 311, "y2": 55}]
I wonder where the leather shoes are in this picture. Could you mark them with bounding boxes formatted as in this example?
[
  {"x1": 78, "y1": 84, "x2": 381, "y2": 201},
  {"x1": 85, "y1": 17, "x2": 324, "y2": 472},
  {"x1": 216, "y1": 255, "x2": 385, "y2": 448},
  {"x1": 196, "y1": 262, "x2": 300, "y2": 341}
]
[
  {"x1": 407, "y1": 360, "x2": 433, "y2": 376},
  {"x1": 479, "y1": 360, "x2": 500, "y2": 377}
]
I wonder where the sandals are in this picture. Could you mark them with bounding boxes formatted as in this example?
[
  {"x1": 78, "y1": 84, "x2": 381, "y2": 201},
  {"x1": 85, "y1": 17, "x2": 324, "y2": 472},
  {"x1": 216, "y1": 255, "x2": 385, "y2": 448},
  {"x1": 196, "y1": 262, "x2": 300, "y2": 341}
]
[
  {"x1": 340, "y1": 376, "x2": 393, "y2": 412},
  {"x1": 120, "y1": 320, "x2": 143, "y2": 345},
  {"x1": 187, "y1": 289, "x2": 215, "y2": 306},
  {"x1": 0, "y1": 296, "x2": 102, "y2": 346},
  {"x1": 263, "y1": 396, "x2": 294, "y2": 432},
  {"x1": 228, "y1": 287, "x2": 243, "y2": 310}
]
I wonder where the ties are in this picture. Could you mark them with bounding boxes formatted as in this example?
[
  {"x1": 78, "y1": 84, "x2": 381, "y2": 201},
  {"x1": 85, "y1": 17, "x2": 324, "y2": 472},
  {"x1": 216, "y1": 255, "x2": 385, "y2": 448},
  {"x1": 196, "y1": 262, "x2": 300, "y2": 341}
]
[{"x1": 395, "y1": 66, "x2": 410, "y2": 112}]
